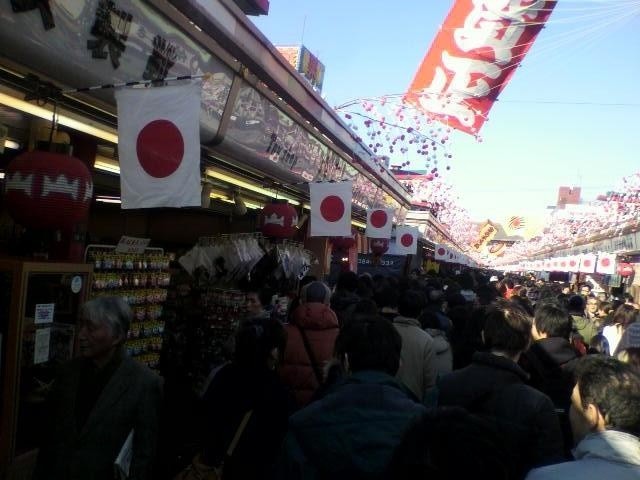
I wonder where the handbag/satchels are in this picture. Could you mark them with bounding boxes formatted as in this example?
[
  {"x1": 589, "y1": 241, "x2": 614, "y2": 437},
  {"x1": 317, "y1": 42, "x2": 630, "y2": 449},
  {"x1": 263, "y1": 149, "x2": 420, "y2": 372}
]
[
  {"x1": 171, "y1": 404, "x2": 254, "y2": 480},
  {"x1": 297, "y1": 322, "x2": 335, "y2": 412}
]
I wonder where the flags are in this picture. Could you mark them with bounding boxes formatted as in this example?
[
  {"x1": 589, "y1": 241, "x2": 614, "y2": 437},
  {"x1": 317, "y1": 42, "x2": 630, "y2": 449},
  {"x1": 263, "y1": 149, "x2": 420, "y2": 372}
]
[
  {"x1": 596, "y1": 254, "x2": 616, "y2": 274},
  {"x1": 568, "y1": 255, "x2": 581, "y2": 272},
  {"x1": 434, "y1": 244, "x2": 446, "y2": 260},
  {"x1": 559, "y1": 256, "x2": 569, "y2": 272},
  {"x1": 579, "y1": 255, "x2": 596, "y2": 273},
  {"x1": 365, "y1": 207, "x2": 393, "y2": 238},
  {"x1": 115, "y1": 80, "x2": 202, "y2": 209},
  {"x1": 310, "y1": 181, "x2": 352, "y2": 236},
  {"x1": 396, "y1": 226, "x2": 417, "y2": 254}
]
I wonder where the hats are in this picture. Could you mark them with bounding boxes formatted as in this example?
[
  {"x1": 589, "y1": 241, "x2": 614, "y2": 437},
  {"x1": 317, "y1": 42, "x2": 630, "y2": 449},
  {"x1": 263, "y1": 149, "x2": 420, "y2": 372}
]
[{"x1": 300, "y1": 281, "x2": 332, "y2": 306}]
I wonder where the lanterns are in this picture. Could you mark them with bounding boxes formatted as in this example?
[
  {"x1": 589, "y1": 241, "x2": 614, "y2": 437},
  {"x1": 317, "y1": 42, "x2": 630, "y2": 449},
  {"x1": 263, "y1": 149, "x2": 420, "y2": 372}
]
[
  {"x1": 370, "y1": 237, "x2": 388, "y2": 257},
  {"x1": 258, "y1": 199, "x2": 298, "y2": 243},
  {"x1": 617, "y1": 261, "x2": 633, "y2": 278},
  {"x1": 335, "y1": 225, "x2": 358, "y2": 254},
  {"x1": 423, "y1": 252, "x2": 432, "y2": 260},
  {"x1": 4, "y1": 141, "x2": 92, "y2": 247}
]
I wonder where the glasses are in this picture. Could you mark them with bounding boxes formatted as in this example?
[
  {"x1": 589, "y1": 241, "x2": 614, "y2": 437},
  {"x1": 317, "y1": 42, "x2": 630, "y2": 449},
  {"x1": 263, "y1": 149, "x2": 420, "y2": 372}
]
[
  {"x1": 589, "y1": 303, "x2": 600, "y2": 308},
  {"x1": 581, "y1": 288, "x2": 589, "y2": 293}
]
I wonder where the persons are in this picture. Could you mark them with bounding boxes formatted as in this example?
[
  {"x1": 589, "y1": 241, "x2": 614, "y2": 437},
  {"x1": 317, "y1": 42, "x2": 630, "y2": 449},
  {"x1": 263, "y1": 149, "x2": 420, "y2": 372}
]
[
  {"x1": 245, "y1": 284, "x2": 274, "y2": 318},
  {"x1": 194, "y1": 315, "x2": 297, "y2": 480},
  {"x1": 33, "y1": 297, "x2": 161, "y2": 480},
  {"x1": 472, "y1": 273, "x2": 640, "y2": 480}
]
